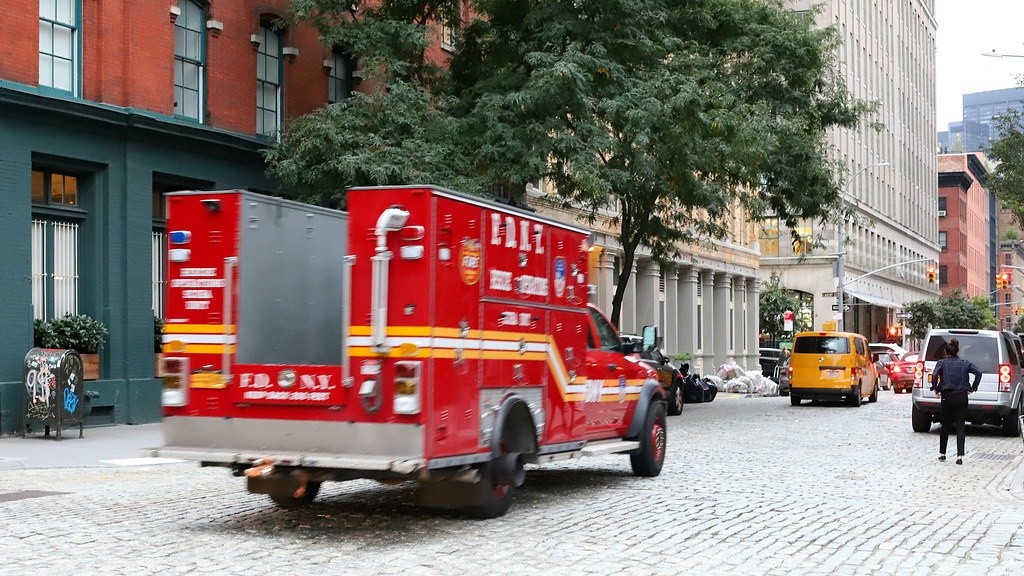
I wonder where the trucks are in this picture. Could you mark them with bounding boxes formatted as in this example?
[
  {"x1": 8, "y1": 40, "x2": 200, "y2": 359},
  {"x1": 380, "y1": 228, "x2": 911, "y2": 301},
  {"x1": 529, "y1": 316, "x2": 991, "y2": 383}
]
[{"x1": 152, "y1": 184, "x2": 668, "y2": 521}]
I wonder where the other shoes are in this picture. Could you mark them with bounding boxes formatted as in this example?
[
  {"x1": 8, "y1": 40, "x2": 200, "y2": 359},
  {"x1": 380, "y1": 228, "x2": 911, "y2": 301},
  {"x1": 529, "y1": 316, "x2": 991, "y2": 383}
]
[
  {"x1": 938, "y1": 456, "x2": 946, "y2": 460},
  {"x1": 956, "y1": 459, "x2": 962, "y2": 464}
]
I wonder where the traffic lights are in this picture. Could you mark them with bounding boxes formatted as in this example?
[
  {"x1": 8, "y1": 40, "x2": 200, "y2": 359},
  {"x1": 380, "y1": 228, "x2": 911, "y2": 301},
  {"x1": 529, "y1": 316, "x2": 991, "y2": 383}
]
[
  {"x1": 1001, "y1": 273, "x2": 1008, "y2": 290},
  {"x1": 887, "y1": 326, "x2": 899, "y2": 338},
  {"x1": 995, "y1": 273, "x2": 1002, "y2": 288},
  {"x1": 927, "y1": 265, "x2": 935, "y2": 283},
  {"x1": 1015, "y1": 308, "x2": 1018, "y2": 315}
]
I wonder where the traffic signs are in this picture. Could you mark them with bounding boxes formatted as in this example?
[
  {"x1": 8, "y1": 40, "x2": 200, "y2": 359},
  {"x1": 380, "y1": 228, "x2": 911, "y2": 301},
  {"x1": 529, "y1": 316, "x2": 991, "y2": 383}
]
[
  {"x1": 895, "y1": 312, "x2": 913, "y2": 319},
  {"x1": 832, "y1": 305, "x2": 851, "y2": 311}
]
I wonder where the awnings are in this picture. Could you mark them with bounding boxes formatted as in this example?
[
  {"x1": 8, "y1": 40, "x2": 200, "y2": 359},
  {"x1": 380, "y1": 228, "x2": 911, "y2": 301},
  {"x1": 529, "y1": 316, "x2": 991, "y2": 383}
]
[{"x1": 843, "y1": 290, "x2": 904, "y2": 309}]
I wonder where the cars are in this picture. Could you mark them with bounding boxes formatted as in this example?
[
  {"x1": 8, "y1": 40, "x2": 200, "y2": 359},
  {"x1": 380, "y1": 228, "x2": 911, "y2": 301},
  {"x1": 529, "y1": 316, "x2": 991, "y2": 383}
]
[
  {"x1": 779, "y1": 356, "x2": 792, "y2": 396},
  {"x1": 868, "y1": 342, "x2": 919, "y2": 394},
  {"x1": 620, "y1": 334, "x2": 686, "y2": 417}
]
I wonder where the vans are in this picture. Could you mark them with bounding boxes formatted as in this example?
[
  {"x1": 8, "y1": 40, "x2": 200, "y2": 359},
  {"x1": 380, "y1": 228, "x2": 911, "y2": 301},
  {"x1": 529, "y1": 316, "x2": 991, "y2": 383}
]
[
  {"x1": 759, "y1": 347, "x2": 790, "y2": 379},
  {"x1": 790, "y1": 330, "x2": 879, "y2": 407}
]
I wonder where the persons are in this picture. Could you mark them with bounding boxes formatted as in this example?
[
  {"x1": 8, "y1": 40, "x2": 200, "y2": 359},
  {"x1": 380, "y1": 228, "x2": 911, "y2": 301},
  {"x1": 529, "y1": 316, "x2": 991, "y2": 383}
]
[
  {"x1": 778, "y1": 345, "x2": 791, "y2": 367},
  {"x1": 930, "y1": 339, "x2": 982, "y2": 465}
]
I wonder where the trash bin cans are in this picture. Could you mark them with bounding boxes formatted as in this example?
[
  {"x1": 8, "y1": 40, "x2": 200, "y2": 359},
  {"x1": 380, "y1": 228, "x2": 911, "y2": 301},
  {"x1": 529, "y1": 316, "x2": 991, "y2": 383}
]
[{"x1": 21, "y1": 347, "x2": 87, "y2": 428}]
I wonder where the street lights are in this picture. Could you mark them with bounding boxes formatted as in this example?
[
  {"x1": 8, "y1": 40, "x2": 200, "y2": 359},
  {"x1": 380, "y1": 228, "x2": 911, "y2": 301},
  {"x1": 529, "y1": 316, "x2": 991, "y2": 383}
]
[{"x1": 837, "y1": 162, "x2": 890, "y2": 332}]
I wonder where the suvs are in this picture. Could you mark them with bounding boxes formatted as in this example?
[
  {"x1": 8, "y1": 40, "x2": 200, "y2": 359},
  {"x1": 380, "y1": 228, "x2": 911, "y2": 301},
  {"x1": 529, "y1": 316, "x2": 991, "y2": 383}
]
[{"x1": 912, "y1": 323, "x2": 1024, "y2": 437}]
